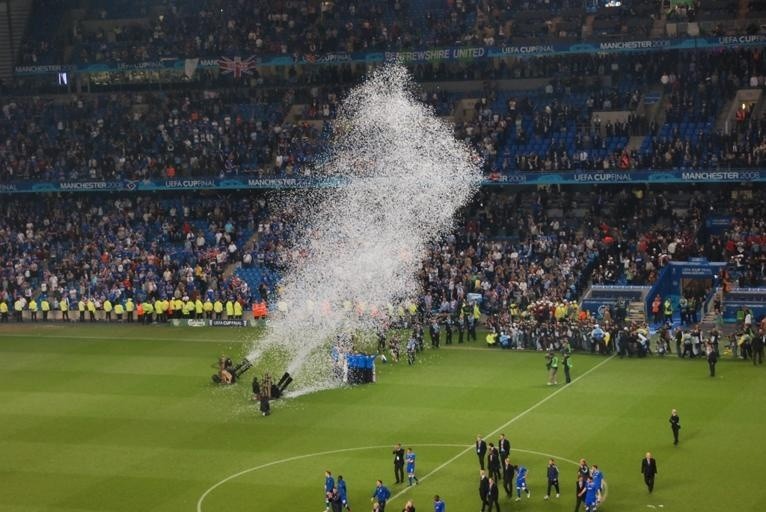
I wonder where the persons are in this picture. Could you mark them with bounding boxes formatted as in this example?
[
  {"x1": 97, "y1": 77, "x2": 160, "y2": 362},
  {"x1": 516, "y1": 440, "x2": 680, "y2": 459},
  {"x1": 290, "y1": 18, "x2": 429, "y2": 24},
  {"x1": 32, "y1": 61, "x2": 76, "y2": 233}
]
[
  {"x1": 641, "y1": 451, "x2": 657, "y2": 494},
  {"x1": 393, "y1": 442, "x2": 419, "y2": 487},
  {"x1": 324, "y1": 470, "x2": 445, "y2": 512},
  {"x1": 543, "y1": 458, "x2": 560, "y2": 501},
  {"x1": 475, "y1": 433, "x2": 531, "y2": 511},
  {"x1": 669, "y1": 408, "x2": 680, "y2": 446},
  {"x1": 575, "y1": 459, "x2": 603, "y2": 512},
  {"x1": 0, "y1": 1, "x2": 766, "y2": 416}
]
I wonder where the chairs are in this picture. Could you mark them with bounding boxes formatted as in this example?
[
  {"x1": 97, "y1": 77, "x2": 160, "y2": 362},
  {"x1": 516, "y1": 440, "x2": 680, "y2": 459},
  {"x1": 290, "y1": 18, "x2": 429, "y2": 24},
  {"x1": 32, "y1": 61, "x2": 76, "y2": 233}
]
[{"x1": 0, "y1": 2, "x2": 766, "y2": 329}]
